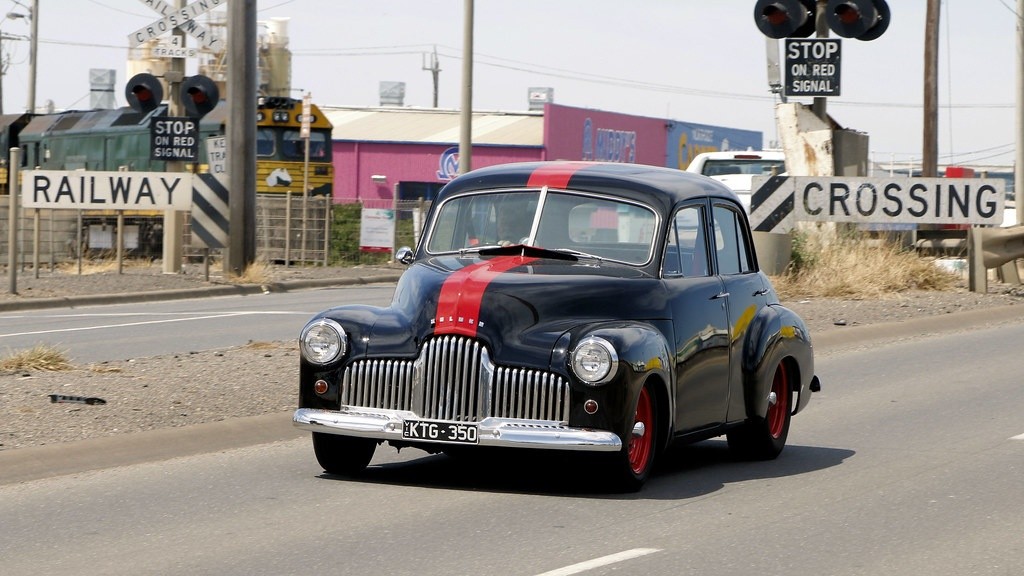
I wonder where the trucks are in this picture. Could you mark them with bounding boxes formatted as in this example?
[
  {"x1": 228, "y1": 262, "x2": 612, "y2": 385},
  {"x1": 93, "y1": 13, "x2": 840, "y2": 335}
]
[{"x1": 617, "y1": 150, "x2": 789, "y2": 249}]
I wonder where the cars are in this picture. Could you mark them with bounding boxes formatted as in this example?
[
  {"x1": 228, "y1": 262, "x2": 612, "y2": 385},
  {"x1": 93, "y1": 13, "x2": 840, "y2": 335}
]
[{"x1": 292, "y1": 159, "x2": 820, "y2": 496}]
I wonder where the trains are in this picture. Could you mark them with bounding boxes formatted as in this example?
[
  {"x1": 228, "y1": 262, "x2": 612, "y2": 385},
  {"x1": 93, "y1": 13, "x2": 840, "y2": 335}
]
[{"x1": 0, "y1": 96, "x2": 333, "y2": 198}]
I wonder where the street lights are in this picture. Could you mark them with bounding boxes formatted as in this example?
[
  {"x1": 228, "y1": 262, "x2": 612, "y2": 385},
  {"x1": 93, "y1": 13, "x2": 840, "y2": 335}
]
[{"x1": 6, "y1": 11, "x2": 39, "y2": 113}]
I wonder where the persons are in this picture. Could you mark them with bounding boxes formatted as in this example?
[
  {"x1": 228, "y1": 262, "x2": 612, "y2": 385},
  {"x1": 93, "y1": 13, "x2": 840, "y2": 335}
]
[{"x1": 494, "y1": 199, "x2": 538, "y2": 247}]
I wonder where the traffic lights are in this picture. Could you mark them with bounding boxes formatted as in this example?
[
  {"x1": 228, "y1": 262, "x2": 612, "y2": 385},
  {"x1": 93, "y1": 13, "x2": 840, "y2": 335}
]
[
  {"x1": 179, "y1": 73, "x2": 218, "y2": 117},
  {"x1": 826, "y1": 0, "x2": 875, "y2": 39},
  {"x1": 754, "y1": 0, "x2": 807, "y2": 39},
  {"x1": 126, "y1": 74, "x2": 165, "y2": 113}
]
